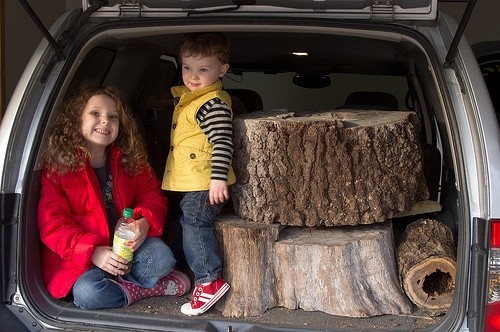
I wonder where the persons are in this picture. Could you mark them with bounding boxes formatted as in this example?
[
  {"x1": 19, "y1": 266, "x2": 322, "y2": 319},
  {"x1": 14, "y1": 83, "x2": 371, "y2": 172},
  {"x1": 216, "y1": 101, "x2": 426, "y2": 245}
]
[
  {"x1": 36, "y1": 83, "x2": 190, "y2": 310},
  {"x1": 162, "y1": 34, "x2": 236, "y2": 315}
]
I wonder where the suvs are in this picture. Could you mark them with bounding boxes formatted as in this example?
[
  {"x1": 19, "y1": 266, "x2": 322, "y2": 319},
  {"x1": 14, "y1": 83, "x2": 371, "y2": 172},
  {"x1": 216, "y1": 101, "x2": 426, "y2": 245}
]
[{"x1": 0, "y1": 1, "x2": 500, "y2": 332}]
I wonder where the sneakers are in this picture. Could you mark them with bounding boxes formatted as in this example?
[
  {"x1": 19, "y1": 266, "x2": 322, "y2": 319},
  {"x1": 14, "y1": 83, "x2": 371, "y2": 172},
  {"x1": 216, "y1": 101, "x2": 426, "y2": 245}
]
[{"x1": 180, "y1": 277, "x2": 231, "y2": 316}]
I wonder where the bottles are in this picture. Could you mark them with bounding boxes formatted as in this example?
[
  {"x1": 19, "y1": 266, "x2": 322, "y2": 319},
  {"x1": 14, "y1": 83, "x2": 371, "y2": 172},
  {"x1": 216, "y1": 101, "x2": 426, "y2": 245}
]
[{"x1": 112, "y1": 208, "x2": 135, "y2": 276}]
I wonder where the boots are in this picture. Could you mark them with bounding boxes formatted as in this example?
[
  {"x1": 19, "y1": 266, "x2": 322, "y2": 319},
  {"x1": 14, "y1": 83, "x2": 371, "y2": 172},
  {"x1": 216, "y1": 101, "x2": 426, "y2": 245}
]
[{"x1": 103, "y1": 271, "x2": 192, "y2": 310}]
